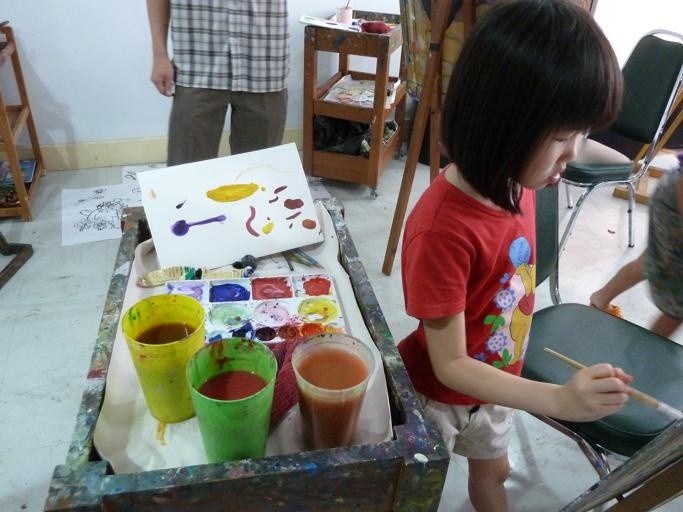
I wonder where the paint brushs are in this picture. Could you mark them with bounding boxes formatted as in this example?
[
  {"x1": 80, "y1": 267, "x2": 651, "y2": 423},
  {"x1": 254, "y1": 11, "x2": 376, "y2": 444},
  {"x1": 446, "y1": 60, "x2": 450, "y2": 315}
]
[{"x1": 544, "y1": 347, "x2": 683, "y2": 423}]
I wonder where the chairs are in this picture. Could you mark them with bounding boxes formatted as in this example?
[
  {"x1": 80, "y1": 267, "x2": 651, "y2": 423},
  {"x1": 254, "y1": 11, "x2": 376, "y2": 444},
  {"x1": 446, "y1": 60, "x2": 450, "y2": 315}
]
[
  {"x1": 519, "y1": 179, "x2": 682, "y2": 511},
  {"x1": 559, "y1": 28, "x2": 682, "y2": 257}
]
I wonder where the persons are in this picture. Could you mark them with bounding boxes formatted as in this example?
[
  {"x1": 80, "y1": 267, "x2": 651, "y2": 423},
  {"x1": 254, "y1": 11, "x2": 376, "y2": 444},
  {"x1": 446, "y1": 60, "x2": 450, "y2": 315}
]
[
  {"x1": 392, "y1": 2, "x2": 635, "y2": 512},
  {"x1": 145, "y1": 0, "x2": 293, "y2": 169},
  {"x1": 588, "y1": 155, "x2": 681, "y2": 336}
]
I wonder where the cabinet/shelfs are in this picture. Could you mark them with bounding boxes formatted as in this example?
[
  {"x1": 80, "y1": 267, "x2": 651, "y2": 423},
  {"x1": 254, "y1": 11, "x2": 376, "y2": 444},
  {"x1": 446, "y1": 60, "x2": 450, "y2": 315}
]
[
  {"x1": 0, "y1": 19, "x2": 47, "y2": 223},
  {"x1": 301, "y1": 5, "x2": 408, "y2": 200}
]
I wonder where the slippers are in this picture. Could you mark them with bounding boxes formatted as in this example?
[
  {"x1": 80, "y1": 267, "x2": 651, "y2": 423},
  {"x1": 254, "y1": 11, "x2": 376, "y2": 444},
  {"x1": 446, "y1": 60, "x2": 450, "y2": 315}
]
[{"x1": 590, "y1": 302, "x2": 621, "y2": 319}]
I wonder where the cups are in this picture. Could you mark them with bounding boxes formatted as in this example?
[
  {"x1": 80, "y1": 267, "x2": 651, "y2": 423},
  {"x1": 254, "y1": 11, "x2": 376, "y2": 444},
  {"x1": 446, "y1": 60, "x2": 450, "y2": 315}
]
[
  {"x1": 289, "y1": 331, "x2": 376, "y2": 449},
  {"x1": 335, "y1": 6, "x2": 352, "y2": 24},
  {"x1": 122, "y1": 295, "x2": 205, "y2": 423},
  {"x1": 185, "y1": 337, "x2": 278, "y2": 460}
]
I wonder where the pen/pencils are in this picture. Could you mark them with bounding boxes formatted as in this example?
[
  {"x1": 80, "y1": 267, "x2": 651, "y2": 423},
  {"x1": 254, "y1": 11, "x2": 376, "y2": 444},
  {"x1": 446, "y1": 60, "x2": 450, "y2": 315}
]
[{"x1": 282, "y1": 247, "x2": 324, "y2": 271}]
